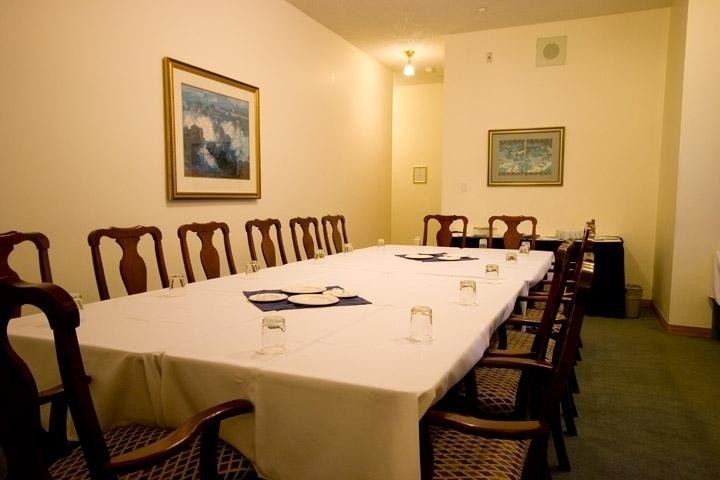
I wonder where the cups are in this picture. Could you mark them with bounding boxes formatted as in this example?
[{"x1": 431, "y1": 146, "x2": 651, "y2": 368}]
[
  {"x1": 70, "y1": 293, "x2": 86, "y2": 324},
  {"x1": 413, "y1": 238, "x2": 422, "y2": 251},
  {"x1": 523, "y1": 242, "x2": 531, "y2": 251},
  {"x1": 479, "y1": 239, "x2": 488, "y2": 251},
  {"x1": 506, "y1": 252, "x2": 518, "y2": 267},
  {"x1": 315, "y1": 249, "x2": 326, "y2": 265},
  {"x1": 245, "y1": 261, "x2": 264, "y2": 285},
  {"x1": 376, "y1": 237, "x2": 385, "y2": 248},
  {"x1": 344, "y1": 243, "x2": 354, "y2": 258},
  {"x1": 260, "y1": 314, "x2": 286, "y2": 355},
  {"x1": 460, "y1": 280, "x2": 478, "y2": 306},
  {"x1": 520, "y1": 245, "x2": 530, "y2": 258},
  {"x1": 485, "y1": 264, "x2": 499, "y2": 285},
  {"x1": 409, "y1": 306, "x2": 434, "y2": 342},
  {"x1": 169, "y1": 274, "x2": 186, "y2": 296}
]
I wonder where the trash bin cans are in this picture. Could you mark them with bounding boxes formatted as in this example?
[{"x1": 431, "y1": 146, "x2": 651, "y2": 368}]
[{"x1": 626, "y1": 284, "x2": 643, "y2": 318}]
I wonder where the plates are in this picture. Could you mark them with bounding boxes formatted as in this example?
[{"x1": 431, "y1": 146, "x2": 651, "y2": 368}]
[
  {"x1": 280, "y1": 285, "x2": 327, "y2": 293},
  {"x1": 405, "y1": 251, "x2": 470, "y2": 260},
  {"x1": 323, "y1": 290, "x2": 359, "y2": 297},
  {"x1": 288, "y1": 294, "x2": 339, "y2": 306},
  {"x1": 248, "y1": 293, "x2": 288, "y2": 302}
]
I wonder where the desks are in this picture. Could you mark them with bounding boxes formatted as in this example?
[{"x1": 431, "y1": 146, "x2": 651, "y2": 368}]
[{"x1": 444, "y1": 233, "x2": 630, "y2": 322}]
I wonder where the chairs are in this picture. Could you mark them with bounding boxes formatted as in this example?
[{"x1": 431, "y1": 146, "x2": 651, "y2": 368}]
[
  {"x1": 290, "y1": 217, "x2": 323, "y2": 262},
  {"x1": 244, "y1": 218, "x2": 289, "y2": 270},
  {"x1": 1, "y1": 282, "x2": 256, "y2": 479},
  {"x1": 321, "y1": 215, "x2": 349, "y2": 254},
  {"x1": 0, "y1": 230, "x2": 67, "y2": 317},
  {"x1": 85, "y1": 224, "x2": 173, "y2": 301},
  {"x1": 178, "y1": 222, "x2": 238, "y2": 283}
]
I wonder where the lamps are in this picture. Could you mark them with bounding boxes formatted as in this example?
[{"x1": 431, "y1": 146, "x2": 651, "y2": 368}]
[{"x1": 403, "y1": 50, "x2": 415, "y2": 76}]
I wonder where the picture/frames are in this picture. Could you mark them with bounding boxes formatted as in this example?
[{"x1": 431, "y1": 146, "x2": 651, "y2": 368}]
[
  {"x1": 161, "y1": 53, "x2": 262, "y2": 202},
  {"x1": 486, "y1": 125, "x2": 565, "y2": 188}
]
